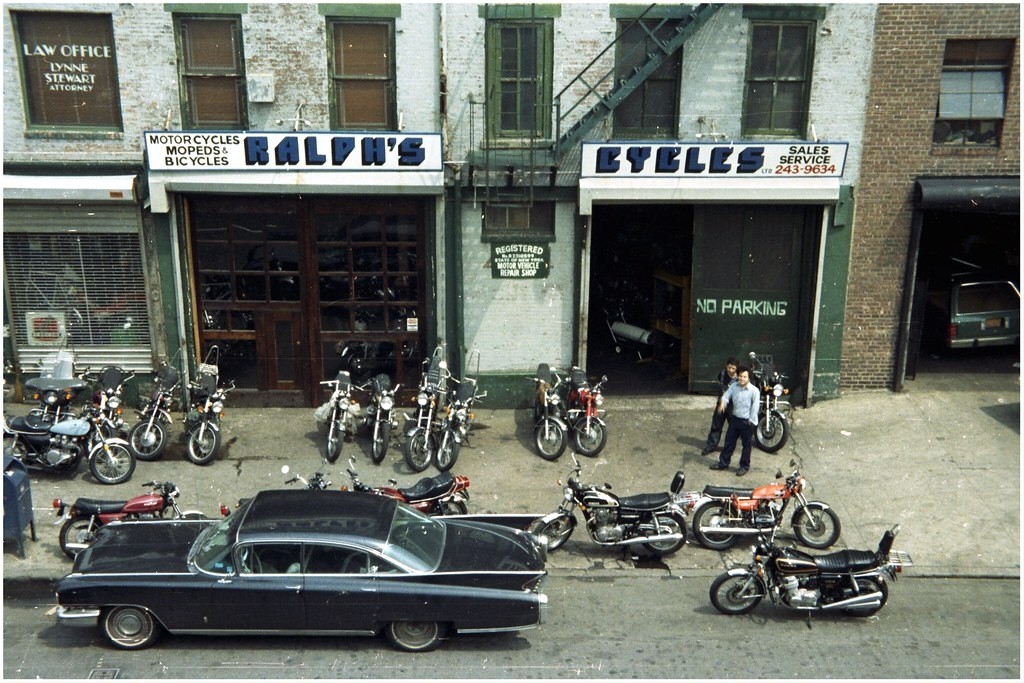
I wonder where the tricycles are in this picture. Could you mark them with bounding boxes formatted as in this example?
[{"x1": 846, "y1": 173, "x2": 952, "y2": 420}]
[{"x1": 217, "y1": 343, "x2": 488, "y2": 518}]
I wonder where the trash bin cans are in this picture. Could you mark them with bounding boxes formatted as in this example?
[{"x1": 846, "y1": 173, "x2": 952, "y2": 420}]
[{"x1": 3, "y1": 447, "x2": 37, "y2": 559}]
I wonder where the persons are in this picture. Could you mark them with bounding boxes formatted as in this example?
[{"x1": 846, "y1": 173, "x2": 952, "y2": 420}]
[
  {"x1": 701, "y1": 356, "x2": 741, "y2": 456},
  {"x1": 709, "y1": 367, "x2": 761, "y2": 476}
]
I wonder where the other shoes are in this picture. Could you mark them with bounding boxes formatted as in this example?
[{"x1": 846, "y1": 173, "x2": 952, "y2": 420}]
[
  {"x1": 710, "y1": 464, "x2": 728, "y2": 470},
  {"x1": 702, "y1": 448, "x2": 714, "y2": 455},
  {"x1": 737, "y1": 468, "x2": 748, "y2": 476}
]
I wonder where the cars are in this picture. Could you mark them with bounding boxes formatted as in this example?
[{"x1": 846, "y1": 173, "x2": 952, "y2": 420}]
[
  {"x1": 925, "y1": 278, "x2": 1020, "y2": 352},
  {"x1": 43, "y1": 488, "x2": 552, "y2": 653},
  {"x1": 199, "y1": 283, "x2": 257, "y2": 369},
  {"x1": 245, "y1": 244, "x2": 398, "y2": 323}
]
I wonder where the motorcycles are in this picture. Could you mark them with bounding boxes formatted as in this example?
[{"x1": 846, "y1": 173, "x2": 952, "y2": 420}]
[
  {"x1": 674, "y1": 447, "x2": 842, "y2": 549},
  {"x1": 709, "y1": 522, "x2": 913, "y2": 629},
  {"x1": 3, "y1": 344, "x2": 235, "y2": 484},
  {"x1": 524, "y1": 361, "x2": 613, "y2": 461},
  {"x1": 50, "y1": 478, "x2": 211, "y2": 560},
  {"x1": 747, "y1": 351, "x2": 794, "y2": 452},
  {"x1": 527, "y1": 453, "x2": 687, "y2": 557}
]
